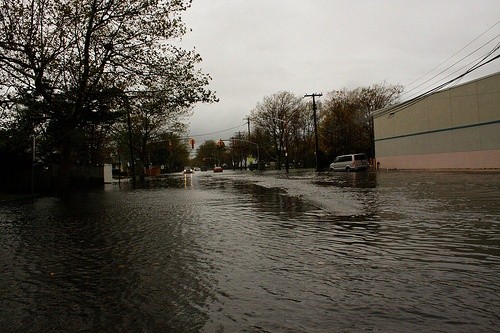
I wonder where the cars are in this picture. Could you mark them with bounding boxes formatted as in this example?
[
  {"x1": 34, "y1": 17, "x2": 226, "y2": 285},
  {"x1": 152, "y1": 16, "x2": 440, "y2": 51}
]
[
  {"x1": 214, "y1": 166, "x2": 223, "y2": 172},
  {"x1": 184, "y1": 167, "x2": 192, "y2": 173}
]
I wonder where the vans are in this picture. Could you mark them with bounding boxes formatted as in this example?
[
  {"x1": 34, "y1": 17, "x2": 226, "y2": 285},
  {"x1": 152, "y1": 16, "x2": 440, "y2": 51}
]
[{"x1": 330, "y1": 154, "x2": 367, "y2": 173}]
